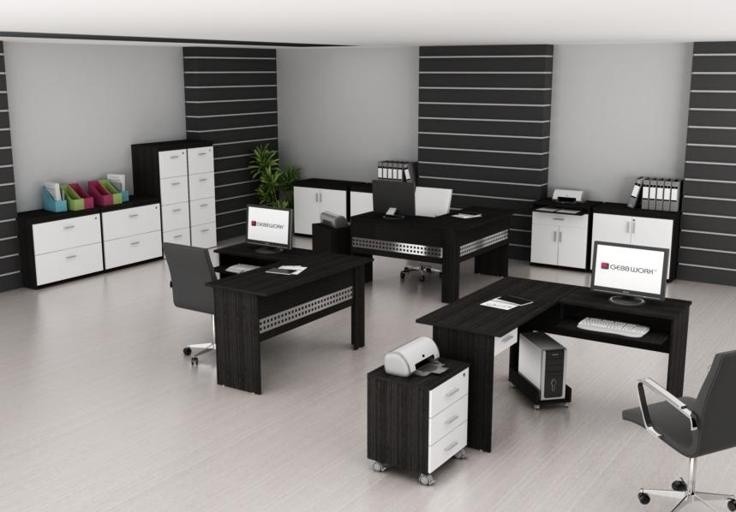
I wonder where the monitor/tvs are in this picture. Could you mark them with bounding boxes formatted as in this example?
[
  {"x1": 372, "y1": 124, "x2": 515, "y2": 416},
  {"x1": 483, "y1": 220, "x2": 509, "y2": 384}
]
[
  {"x1": 591, "y1": 240, "x2": 669, "y2": 306},
  {"x1": 246, "y1": 204, "x2": 294, "y2": 254},
  {"x1": 372, "y1": 179, "x2": 416, "y2": 221}
]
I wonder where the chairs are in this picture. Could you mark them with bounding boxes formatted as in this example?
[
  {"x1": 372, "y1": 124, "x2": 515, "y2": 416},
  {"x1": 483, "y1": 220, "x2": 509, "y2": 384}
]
[
  {"x1": 161, "y1": 242, "x2": 232, "y2": 367},
  {"x1": 621, "y1": 348, "x2": 735, "y2": 511}
]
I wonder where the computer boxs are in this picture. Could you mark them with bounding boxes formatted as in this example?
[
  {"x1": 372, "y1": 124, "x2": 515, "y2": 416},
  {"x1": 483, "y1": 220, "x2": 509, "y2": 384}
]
[{"x1": 518, "y1": 333, "x2": 567, "y2": 402}]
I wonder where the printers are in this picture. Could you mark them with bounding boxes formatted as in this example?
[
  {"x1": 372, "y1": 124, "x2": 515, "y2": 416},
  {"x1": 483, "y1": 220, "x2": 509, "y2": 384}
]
[
  {"x1": 552, "y1": 188, "x2": 585, "y2": 205},
  {"x1": 384, "y1": 336, "x2": 449, "y2": 378},
  {"x1": 320, "y1": 208, "x2": 350, "y2": 229}
]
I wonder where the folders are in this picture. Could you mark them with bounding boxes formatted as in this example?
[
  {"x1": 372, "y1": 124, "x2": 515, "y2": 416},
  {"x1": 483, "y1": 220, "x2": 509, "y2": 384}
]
[
  {"x1": 377, "y1": 160, "x2": 418, "y2": 186},
  {"x1": 627, "y1": 176, "x2": 684, "y2": 212}
]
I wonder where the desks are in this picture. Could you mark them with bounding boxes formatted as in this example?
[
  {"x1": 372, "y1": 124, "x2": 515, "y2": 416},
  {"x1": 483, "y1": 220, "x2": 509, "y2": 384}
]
[
  {"x1": 350, "y1": 205, "x2": 517, "y2": 303},
  {"x1": 416, "y1": 277, "x2": 693, "y2": 453},
  {"x1": 205, "y1": 242, "x2": 374, "y2": 396}
]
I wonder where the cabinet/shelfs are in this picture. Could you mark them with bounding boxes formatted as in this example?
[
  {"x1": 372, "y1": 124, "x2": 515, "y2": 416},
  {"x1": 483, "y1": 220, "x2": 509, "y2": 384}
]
[
  {"x1": 99, "y1": 195, "x2": 163, "y2": 273},
  {"x1": 588, "y1": 201, "x2": 681, "y2": 284},
  {"x1": 529, "y1": 196, "x2": 591, "y2": 273},
  {"x1": 366, "y1": 352, "x2": 470, "y2": 488},
  {"x1": 349, "y1": 183, "x2": 374, "y2": 218},
  {"x1": 132, "y1": 139, "x2": 217, "y2": 260},
  {"x1": 17, "y1": 208, "x2": 103, "y2": 289},
  {"x1": 292, "y1": 178, "x2": 350, "y2": 238},
  {"x1": 312, "y1": 221, "x2": 372, "y2": 283}
]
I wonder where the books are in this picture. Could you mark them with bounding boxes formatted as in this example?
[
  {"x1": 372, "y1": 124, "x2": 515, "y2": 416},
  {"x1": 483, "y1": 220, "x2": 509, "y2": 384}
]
[
  {"x1": 45, "y1": 182, "x2": 61, "y2": 201},
  {"x1": 106, "y1": 173, "x2": 126, "y2": 192},
  {"x1": 627, "y1": 177, "x2": 681, "y2": 210},
  {"x1": 378, "y1": 159, "x2": 415, "y2": 181}
]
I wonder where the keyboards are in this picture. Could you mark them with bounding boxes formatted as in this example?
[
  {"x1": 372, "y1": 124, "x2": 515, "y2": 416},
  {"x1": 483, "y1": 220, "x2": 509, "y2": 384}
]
[
  {"x1": 225, "y1": 263, "x2": 263, "y2": 275},
  {"x1": 577, "y1": 317, "x2": 650, "y2": 338}
]
